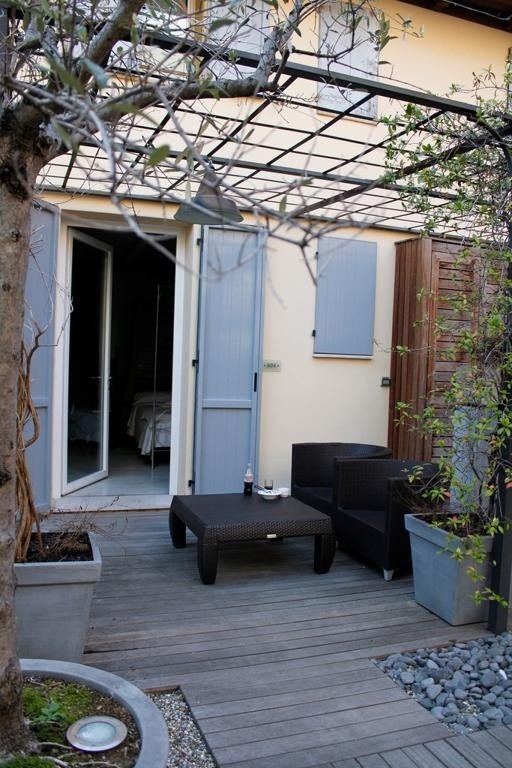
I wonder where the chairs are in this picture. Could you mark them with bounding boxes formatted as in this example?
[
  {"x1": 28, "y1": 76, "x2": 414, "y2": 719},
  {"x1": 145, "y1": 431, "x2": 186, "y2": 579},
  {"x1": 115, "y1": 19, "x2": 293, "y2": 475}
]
[
  {"x1": 334, "y1": 457, "x2": 451, "y2": 582},
  {"x1": 289, "y1": 441, "x2": 395, "y2": 530}
]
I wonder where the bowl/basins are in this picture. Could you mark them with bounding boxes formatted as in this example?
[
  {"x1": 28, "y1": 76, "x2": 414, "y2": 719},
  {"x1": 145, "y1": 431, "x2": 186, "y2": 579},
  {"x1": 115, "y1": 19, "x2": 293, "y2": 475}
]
[{"x1": 257, "y1": 489, "x2": 282, "y2": 500}]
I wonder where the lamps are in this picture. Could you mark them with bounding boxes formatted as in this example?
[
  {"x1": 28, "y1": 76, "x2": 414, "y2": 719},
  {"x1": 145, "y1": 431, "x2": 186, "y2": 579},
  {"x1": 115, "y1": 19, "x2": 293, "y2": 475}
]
[{"x1": 173, "y1": 157, "x2": 246, "y2": 227}]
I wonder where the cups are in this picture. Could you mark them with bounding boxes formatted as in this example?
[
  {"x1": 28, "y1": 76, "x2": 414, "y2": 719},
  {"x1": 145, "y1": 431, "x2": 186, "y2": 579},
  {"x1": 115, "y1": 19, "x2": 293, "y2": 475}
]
[
  {"x1": 264, "y1": 479, "x2": 273, "y2": 491},
  {"x1": 277, "y1": 487, "x2": 291, "y2": 499}
]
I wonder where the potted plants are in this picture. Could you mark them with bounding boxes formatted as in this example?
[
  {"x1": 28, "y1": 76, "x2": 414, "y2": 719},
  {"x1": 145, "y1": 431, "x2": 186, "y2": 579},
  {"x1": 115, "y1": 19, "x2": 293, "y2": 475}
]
[{"x1": 372, "y1": 48, "x2": 512, "y2": 629}]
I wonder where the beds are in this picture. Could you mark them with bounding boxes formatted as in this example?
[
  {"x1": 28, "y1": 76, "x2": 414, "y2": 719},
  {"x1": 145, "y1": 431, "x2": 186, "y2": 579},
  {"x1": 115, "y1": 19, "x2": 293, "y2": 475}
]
[{"x1": 126, "y1": 385, "x2": 171, "y2": 471}]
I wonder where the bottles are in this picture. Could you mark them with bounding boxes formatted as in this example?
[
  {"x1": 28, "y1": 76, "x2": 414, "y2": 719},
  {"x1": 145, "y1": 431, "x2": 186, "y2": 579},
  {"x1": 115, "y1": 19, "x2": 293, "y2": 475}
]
[{"x1": 243, "y1": 462, "x2": 253, "y2": 497}]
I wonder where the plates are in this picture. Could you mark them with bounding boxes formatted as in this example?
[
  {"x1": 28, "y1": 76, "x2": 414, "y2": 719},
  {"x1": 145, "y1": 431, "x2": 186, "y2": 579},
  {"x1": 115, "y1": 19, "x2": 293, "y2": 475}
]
[{"x1": 66, "y1": 715, "x2": 128, "y2": 753}]
